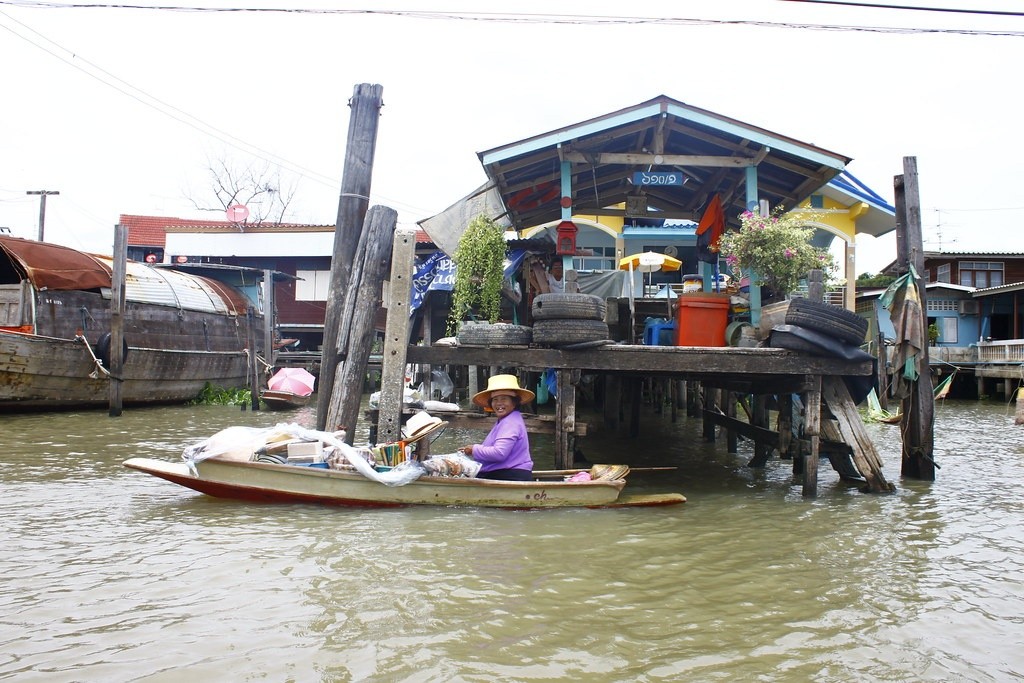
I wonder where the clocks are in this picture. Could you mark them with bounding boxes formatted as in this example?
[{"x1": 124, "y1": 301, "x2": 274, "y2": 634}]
[{"x1": 625, "y1": 195, "x2": 647, "y2": 216}]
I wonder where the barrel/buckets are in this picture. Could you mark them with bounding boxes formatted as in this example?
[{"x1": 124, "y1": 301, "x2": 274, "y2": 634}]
[{"x1": 679, "y1": 296, "x2": 731, "y2": 347}]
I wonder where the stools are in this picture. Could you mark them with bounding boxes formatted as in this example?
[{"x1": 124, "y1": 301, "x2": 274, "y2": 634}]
[{"x1": 642, "y1": 318, "x2": 675, "y2": 347}]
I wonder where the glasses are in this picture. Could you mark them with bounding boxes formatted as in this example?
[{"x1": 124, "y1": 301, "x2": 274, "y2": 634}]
[{"x1": 554, "y1": 265, "x2": 562, "y2": 269}]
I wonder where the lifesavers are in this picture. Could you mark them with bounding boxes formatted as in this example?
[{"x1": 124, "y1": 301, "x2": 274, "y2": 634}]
[
  {"x1": 94, "y1": 330, "x2": 130, "y2": 372},
  {"x1": 456, "y1": 292, "x2": 610, "y2": 346},
  {"x1": 783, "y1": 296, "x2": 870, "y2": 346}
]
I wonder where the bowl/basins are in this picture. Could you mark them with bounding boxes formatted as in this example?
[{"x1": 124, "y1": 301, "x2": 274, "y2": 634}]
[{"x1": 724, "y1": 321, "x2": 751, "y2": 346}]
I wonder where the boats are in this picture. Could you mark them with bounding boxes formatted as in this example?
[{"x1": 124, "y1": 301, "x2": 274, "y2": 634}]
[
  {"x1": 122, "y1": 427, "x2": 690, "y2": 509},
  {"x1": 0, "y1": 238, "x2": 250, "y2": 411},
  {"x1": 259, "y1": 390, "x2": 311, "y2": 410}
]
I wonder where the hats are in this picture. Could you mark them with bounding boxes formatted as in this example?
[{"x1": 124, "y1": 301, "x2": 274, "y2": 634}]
[
  {"x1": 402, "y1": 410, "x2": 449, "y2": 445},
  {"x1": 471, "y1": 374, "x2": 535, "y2": 407}
]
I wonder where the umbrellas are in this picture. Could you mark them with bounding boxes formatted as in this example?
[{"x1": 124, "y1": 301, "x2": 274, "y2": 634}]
[
  {"x1": 618, "y1": 250, "x2": 683, "y2": 297},
  {"x1": 267, "y1": 368, "x2": 316, "y2": 396}
]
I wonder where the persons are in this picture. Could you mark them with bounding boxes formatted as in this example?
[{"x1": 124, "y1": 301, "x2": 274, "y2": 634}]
[
  {"x1": 458, "y1": 374, "x2": 535, "y2": 481},
  {"x1": 545, "y1": 258, "x2": 562, "y2": 294}
]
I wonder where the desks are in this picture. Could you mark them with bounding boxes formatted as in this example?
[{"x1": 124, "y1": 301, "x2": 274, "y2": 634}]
[{"x1": 617, "y1": 296, "x2": 674, "y2": 343}]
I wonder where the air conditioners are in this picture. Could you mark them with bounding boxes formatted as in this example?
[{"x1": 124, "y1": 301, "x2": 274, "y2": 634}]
[{"x1": 959, "y1": 300, "x2": 979, "y2": 315}]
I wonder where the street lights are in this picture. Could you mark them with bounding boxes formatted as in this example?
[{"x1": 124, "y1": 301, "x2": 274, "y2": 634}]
[{"x1": 26, "y1": 190, "x2": 62, "y2": 241}]
